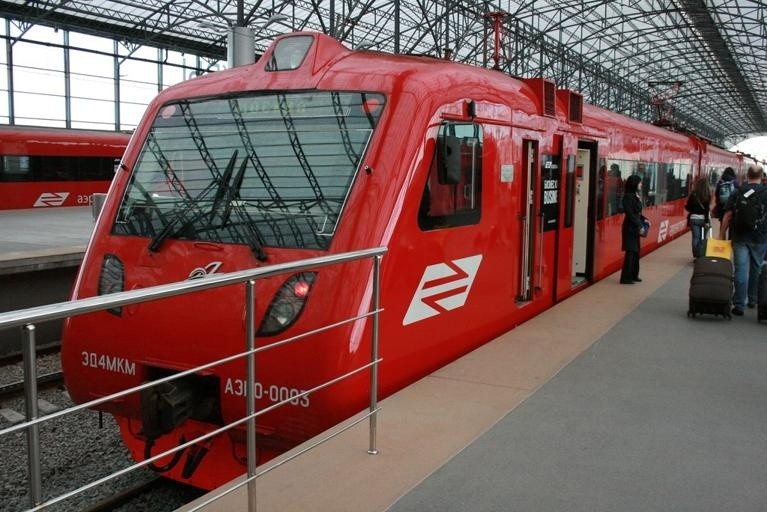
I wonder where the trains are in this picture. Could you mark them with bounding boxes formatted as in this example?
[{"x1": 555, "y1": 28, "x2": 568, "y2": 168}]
[
  {"x1": 0, "y1": 124, "x2": 135, "y2": 210},
  {"x1": 61, "y1": 31, "x2": 767, "y2": 492}
]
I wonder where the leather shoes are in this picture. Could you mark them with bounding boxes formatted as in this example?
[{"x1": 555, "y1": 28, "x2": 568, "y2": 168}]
[
  {"x1": 620, "y1": 275, "x2": 642, "y2": 285},
  {"x1": 731, "y1": 301, "x2": 756, "y2": 316}
]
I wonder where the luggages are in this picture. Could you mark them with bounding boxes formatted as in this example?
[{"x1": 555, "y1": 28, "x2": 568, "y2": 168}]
[
  {"x1": 693, "y1": 238, "x2": 707, "y2": 257},
  {"x1": 685, "y1": 256, "x2": 734, "y2": 320},
  {"x1": 757, "y1": 262, "x2": 767, "y2": 325}
]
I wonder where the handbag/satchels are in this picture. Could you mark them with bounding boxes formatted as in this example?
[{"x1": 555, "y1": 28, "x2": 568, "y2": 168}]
[{"x1": 639, "y1": 221, "x2": 650, "y2": 238}]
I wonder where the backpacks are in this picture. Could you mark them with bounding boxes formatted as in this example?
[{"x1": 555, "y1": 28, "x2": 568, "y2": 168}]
[
  {"x1": 735, "y1": 187, "x2": 767, "y2": 237},
  {"x1": 719, "y1": 181, "x2": 735, "y2": 211}
]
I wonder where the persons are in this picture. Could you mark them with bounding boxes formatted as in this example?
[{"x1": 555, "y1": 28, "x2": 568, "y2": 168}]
[
  {"x1": 620, "y1": 176, "x2": 650, "y2": 284},
  {"x1": 679, "y1": 163, "x2": 766, "y2": 316},
  {"x1": 589, "y1": 157, "x2": 717, "y2": 216}
]
